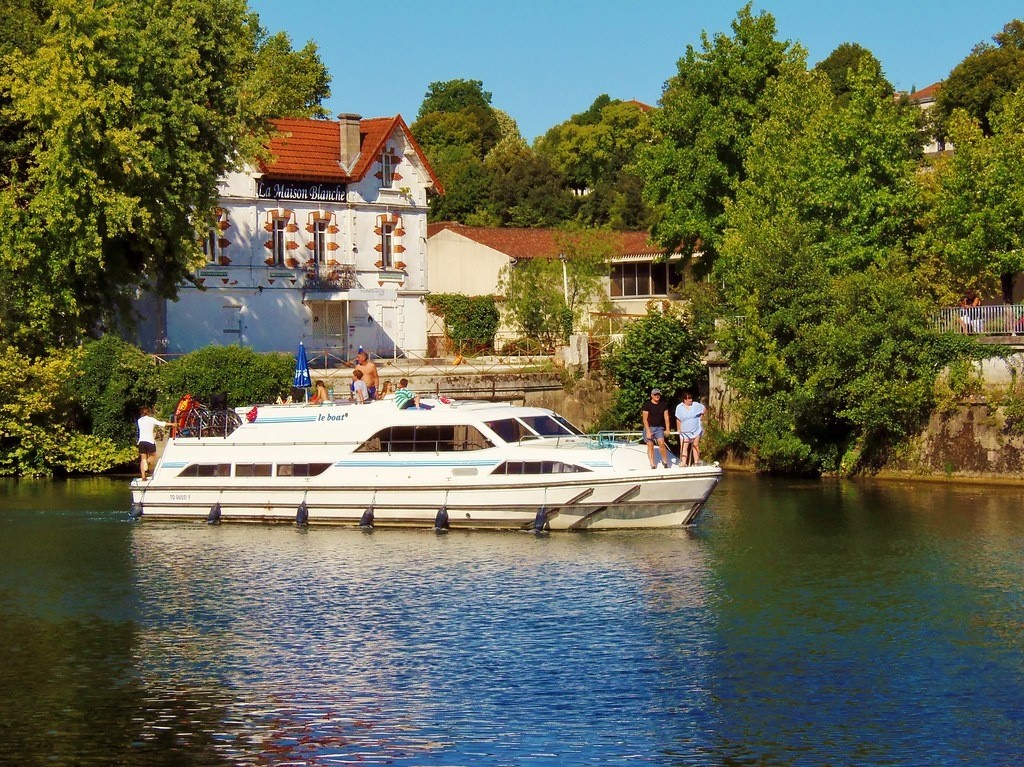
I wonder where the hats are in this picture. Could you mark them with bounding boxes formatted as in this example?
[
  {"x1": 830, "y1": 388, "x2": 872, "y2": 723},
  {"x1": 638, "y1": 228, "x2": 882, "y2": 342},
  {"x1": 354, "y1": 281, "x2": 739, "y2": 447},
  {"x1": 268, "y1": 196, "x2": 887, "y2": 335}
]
[{"x1": 652, "y1": 388, "x2": 662, "y2": 395}]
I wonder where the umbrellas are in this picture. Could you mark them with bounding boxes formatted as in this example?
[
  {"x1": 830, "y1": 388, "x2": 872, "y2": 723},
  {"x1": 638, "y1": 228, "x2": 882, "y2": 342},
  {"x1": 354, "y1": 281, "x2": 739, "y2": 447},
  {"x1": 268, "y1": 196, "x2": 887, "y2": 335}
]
[{"x1": 292, "y1": 342, "x2": 312, "y2": 403}]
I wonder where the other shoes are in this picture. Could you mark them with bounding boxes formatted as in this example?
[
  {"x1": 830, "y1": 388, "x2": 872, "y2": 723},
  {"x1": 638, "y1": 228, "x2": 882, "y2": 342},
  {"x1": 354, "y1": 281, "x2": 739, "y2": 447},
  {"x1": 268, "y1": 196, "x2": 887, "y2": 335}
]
[
  {"x1": 142, "y1": 478, "x2": 147, "y2": 481},
  {"x1": 144, "y1": 459, "x2": 149, "y2": 471}
]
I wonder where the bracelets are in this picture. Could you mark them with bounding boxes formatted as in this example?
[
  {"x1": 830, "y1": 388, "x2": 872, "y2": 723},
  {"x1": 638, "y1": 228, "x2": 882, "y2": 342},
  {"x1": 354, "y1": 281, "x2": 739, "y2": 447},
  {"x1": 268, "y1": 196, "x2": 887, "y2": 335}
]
[{"x1": 376, "y1": 389, "x2": 378, "y2": 392}]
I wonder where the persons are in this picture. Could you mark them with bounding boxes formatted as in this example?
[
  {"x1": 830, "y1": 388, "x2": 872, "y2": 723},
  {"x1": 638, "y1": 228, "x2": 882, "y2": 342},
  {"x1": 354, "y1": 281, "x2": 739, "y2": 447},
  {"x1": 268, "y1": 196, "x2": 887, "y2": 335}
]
[
  {"x1": 381, "y1": 381, "x2": 394, "y2": 399},
  {"x1": 353, "y1": 370, "x2": 369, "y2": 405},
  {"x1": 314, "y1": 380, "x2": 331, "y2": 407},
  {"x1": 642, "y1": 387, "x2": 670, "y2": 469},
  {"x1": 675, "y1": 393, "x2": 707, "y2": 466},
  {"x1": 355, "y1": 351, "x2": 379, "y2": 400},
  {"x1": 395, "y1": 378, "x2": 434, "y2": 409},
  {"x1": 138, "y1": 407, "x2": 178, "y2": 481},
  {"x1": 957, "y1": 293, "x2": 984, "y2": 336}
]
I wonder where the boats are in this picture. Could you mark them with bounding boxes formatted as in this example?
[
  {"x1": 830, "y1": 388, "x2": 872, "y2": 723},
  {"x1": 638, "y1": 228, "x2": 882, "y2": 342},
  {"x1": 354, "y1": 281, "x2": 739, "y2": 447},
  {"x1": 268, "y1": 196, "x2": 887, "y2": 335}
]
[{"x1": 127, "y1": 394, "x2": 724, "y2": 534}]
